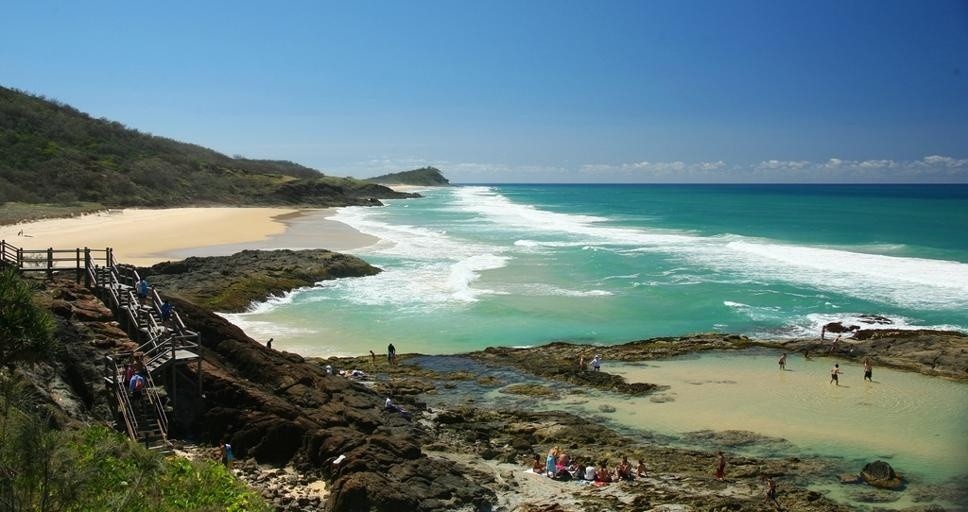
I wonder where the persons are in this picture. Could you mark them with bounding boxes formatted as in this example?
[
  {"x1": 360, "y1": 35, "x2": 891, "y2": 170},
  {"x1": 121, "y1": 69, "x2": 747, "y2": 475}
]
[
  {"x1": 130, "y1": 371, "x2": 145, "y2": 410},
  {"x1": 778, "y1": 325, "x2": 872, "y2": 386},
  {"x1": 589, "y1": 355, "x2": 600, "y2": 372},
  {"x1": 160, "y1": 301, "x2": 173, "y2": 325},
  {"x1": 135, "y1": 277, "x2": 148, "y2": 307},
  {"x1": 267, "y1": 338, "x2": 273, "y2": 348},
  {"x1": 385, "y1": 395, "x2": 398, "y2": 411},
  {"x1": 370, "y1": 350, "x2": 375, "y2": 365},
  {"x1": 762, "y1": 476, "x2": 776, "y2": 503},
  {"x1": 579, "y1": 355, "x2": 585, "y2": 372},
  {"x1": 388, "y1": 343, "x2": 395, "y2": 364},
  {"x1": 532, "y1": 445, "x2": 649, "y2": 482},
  {"x1": 122, "y1": 363, "x2": 135, "y2": 388},
  {"x1": 718, "y1": 452, "x2": 726, "y2": 477},
  {"x1": 212, "y1": 440, "x2": 233, "y2": 467}
]
